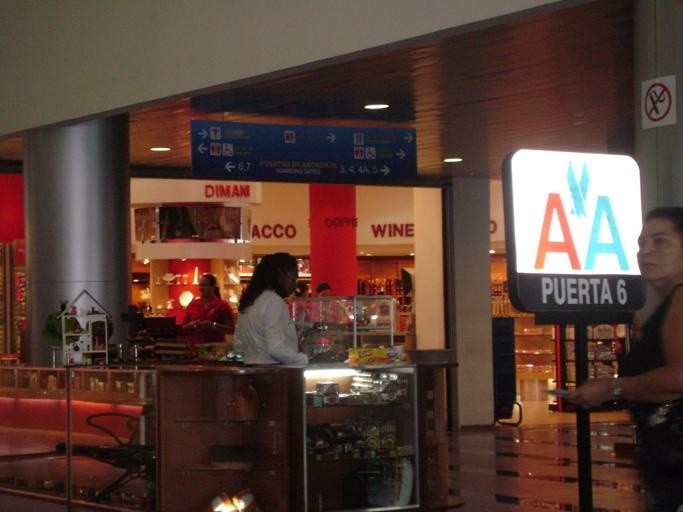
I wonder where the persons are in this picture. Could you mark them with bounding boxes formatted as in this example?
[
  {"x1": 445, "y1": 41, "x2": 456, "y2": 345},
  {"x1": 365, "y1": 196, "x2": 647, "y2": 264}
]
[
  {"x1": 180, "y1": 274, "x2": 235, "y2": 344},
  {"x1": 235, "y1": 252, "x2": 309, "y2": 366},
  {"x1": 568, "y1": 206, "x2": 683, "y2": 511},
  {"x1": 311, "y1": 284, "x2": 340, "y2": 324},
  {"x1": 287, "y1": 283, "x2": 311, "y2": 324}
]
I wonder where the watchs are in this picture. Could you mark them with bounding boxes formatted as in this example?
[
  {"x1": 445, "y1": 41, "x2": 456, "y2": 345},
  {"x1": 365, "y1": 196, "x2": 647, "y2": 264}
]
[{"x1": 611, "y1": 377, "x2": 623, "y2": 403}]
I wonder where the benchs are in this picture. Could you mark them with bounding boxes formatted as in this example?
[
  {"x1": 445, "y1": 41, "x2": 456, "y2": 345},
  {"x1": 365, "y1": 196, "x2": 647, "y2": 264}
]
[{"x1": 2, "y1": 390, "x2": 156, "y2": 510}]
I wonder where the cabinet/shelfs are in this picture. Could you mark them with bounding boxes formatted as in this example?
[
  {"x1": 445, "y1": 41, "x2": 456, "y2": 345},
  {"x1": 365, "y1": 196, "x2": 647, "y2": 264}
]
[
  {"x1": 130, "y1": 250, "x2": 168, "y2": 315},
  {"x1": 511, "y1": 313, "x2": 554, "y2": 403},
  {"x1": 157, "y1": 363, "x2": 292, "y2": 512},
  {"x1": 59, "y1": 313, "x2": 108, "y2": 368},
  {"x1": 298, "y1": 363, "x2": 422, "y2": 512},
  {"x1": 296, "y1": 294, "x2": 396, "y2": 350},
  {"x1": 152, "y1": 254, "x2": 219, "y2": 313},
  {"x1": 548, "y1": 318, "x2": 629, "y2": 412}
]
